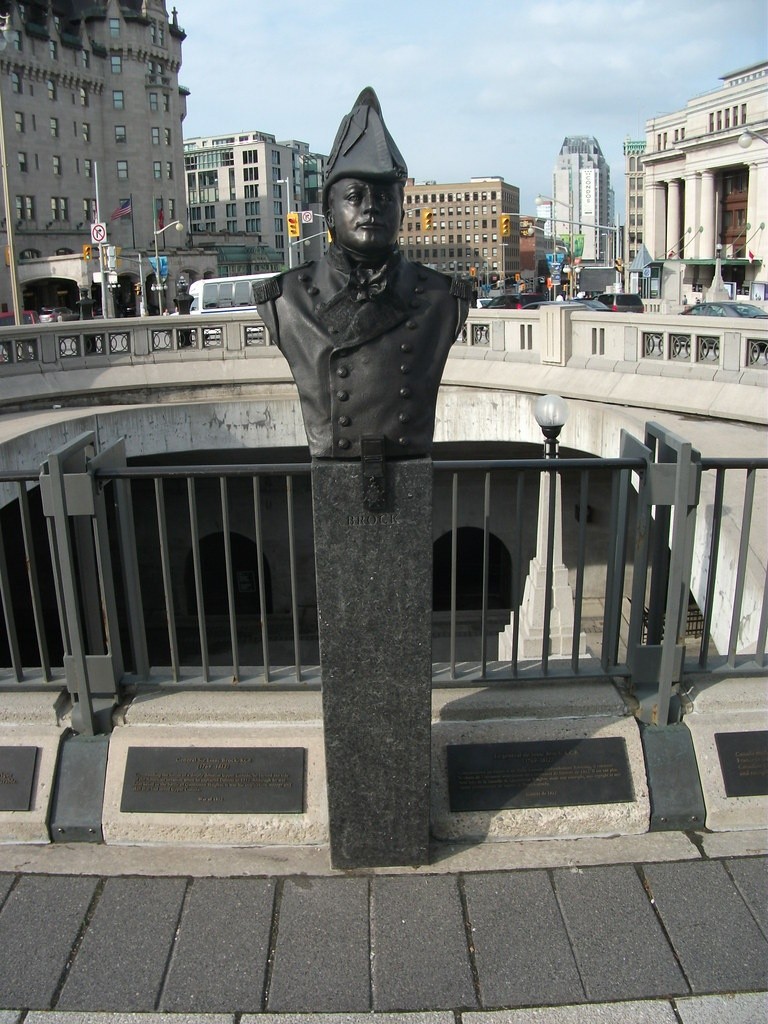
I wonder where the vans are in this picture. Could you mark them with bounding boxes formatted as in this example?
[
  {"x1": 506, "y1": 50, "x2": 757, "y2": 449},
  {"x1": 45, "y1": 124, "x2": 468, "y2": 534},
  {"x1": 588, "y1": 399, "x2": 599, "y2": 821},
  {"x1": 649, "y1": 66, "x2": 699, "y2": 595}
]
[{"x1": 189, "y1": 272, "x2": 286, "y2": 340}]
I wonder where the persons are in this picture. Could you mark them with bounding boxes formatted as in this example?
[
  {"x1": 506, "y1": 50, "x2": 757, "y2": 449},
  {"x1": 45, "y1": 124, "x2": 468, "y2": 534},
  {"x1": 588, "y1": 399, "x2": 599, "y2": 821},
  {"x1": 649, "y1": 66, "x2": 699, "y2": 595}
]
[
  {"x1": 172, "y1": 284, "x2": 194, "y2": 312},
  {"x1": 163, "y1": 308, "x2": 169, "y2": 316},
  {"x1": 57, "y1": 312, "x2": 63, "y2": 322},
  {"x1": 75, "y1": 288, "x2": 96, "y2": 315},
  {"x1": 695, "y1": 297, "x2": 700, "y2": 304},
  {"x1": 682, "y1": 295, "x2": 687, "y2": 305},
  {"x1": 252, "y1": 86, "x2": 473, "y2": 458}
]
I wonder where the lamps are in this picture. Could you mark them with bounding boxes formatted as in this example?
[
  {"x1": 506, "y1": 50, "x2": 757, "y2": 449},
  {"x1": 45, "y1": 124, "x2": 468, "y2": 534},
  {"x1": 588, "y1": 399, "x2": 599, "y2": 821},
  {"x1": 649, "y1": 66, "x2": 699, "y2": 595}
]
[
  {"x1": 46, "y1": 221, "x2": 53, "y2": 230},
  {"x1": 76, "y1": 222, "x2": 83, "y2": 230},
  {"x1": 16, "y1": 220, "x2": 23, "y2": 230}
]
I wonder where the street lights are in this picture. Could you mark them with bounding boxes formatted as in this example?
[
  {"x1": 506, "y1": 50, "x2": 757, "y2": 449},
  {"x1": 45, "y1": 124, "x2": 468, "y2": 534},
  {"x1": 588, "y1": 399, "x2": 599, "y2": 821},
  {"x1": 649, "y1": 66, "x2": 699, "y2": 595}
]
[
  {"x1": 534, "y1": 192, "x2": 574, "y2": 304},
  {"x1": 276, "y1": 176, "x2": 292, "y2": 268},
  {"x1": 150, "y1": 219, "x2": 184, "y2": 315},
  {"x1": 527, "y1": 225, "x2": 556, "y2": 302}
]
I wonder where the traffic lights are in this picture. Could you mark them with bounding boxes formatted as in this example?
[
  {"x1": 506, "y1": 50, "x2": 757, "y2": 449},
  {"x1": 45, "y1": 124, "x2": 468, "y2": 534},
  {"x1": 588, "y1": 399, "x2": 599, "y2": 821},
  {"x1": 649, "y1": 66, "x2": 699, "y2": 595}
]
[
  {"x1": 108, "y1": 246, "x2": 122, "y2": 269},
  {"x1": 136, "y1": 291, "x2": 142, "y2": 296},
  {"x1": 515, "y1": 274, "x2": 520, "y2": 282},
  {"x1": 471, "y1": 267, "x2": 475, "y2": 277},
  {"x1": 83, "y1": 244, "x2": 93, "y2": 264},
  {"x1": 421, "y1": 208, "x2": 434, "y2": 234},
  {"x1": 134, "y1": 281, "x2": 140, "y2": 291},
  {"x1": 613, "y1": 259, "x2": 622, "y2": 272},
  {"x1": 288, "y1": 213, "x2": 299, "y2": 237},
  {"x1": 500, "y1": 214, "x2": 510, "y2": 237}
]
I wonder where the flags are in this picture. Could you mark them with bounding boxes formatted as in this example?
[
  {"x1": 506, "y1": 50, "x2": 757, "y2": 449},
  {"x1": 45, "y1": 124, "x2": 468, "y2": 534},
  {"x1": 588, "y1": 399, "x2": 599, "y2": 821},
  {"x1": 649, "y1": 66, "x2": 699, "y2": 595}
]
[
  {"x1": 749, "y1": 250, "x2": 754, "y2": 264},
  {"x1": 158, "y1": 208, "x2": 164, "y2": 230},
  {"x1": 668, "y1": 251, "x2": 674, "y2": 259},
  {"x1": 111, "y1": 199, "x2": 132, "y2": 221}
]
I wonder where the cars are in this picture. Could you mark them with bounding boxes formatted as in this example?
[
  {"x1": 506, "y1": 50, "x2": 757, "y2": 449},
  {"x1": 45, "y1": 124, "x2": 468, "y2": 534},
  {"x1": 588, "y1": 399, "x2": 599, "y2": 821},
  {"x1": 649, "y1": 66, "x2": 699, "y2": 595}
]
[
  {"x1": 653, "y1": 301, "x2": 768, "y2": 354},
  {"x1": 519, "y1": 300, "x2": 612, "y2": 311},
  {"x1": 123, "y1": 304, "x2": 159, "y2": 317},
  {"x1": 479, "y1": 293, "x2": 546, "y2": 309},
  {"x1": 476, "y1": 298, "x2": 494, "y2": 309},
  {"x1": 0, "y1": 305, "x2": 81, "y2": 327}
]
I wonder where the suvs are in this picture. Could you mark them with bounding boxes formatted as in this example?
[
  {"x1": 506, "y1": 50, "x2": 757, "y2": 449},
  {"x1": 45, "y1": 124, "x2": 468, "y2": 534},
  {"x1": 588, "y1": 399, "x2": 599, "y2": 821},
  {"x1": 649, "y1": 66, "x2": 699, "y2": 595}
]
[{"x1": 592, "y1": 292, "x2": 645, "y2": 313}]
